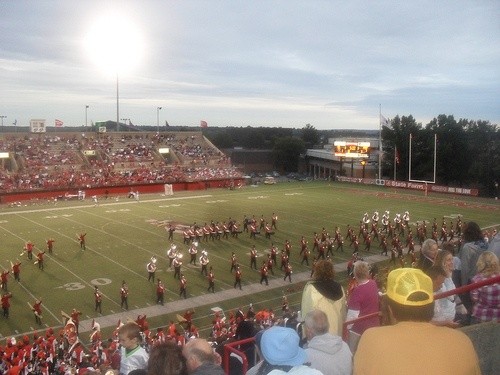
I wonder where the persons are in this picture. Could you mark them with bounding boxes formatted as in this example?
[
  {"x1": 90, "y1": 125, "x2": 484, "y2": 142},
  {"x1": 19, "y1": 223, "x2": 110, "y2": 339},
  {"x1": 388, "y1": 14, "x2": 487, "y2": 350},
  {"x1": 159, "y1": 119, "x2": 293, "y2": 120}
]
[
  {"x1": 0, "y1": 292, "x2": 304, "y2": 375},
  {"x1": 418, "y1": 222, "x2": 500, "y2": 328},
  {"x1": 349, "y1": 260, "x2": 380, "y2": 335},
  {"x1": 0, "y1": 232, "x2": 129, "y2": 325},
  {"x1": 0, "y1": 132, "x2": 243, "y2": 194},
  {"x1": 183, "y1": 337, "x2": 225, "y2": 375},
  {"x1": 167, "y1": 212, "x2": 278, "y2": 246},
  {"x1": 313, "y1": 211, "x2": 461, "y2": 268},
  {"x1": 9, "y1": 187, "x2": 136, "y2": 208},
  {"x1": 353, "y1": 268, "x2": 480, "y2": 375},
  {"x1": 146, "y1": 235, "x2": 310, "y2": 306},
  {"x1": 118, "y1": 322, "x2": 149, "y2": 375},
  {"x1": 128, "y1": 341, "x2": 222, "y2": 375},
  {"x1": 304, "y1": 309, "x2": 352, "y2": 375},
  {"x1": 254, "y1": 326, "x2": 323, "y2": 375},
  {"x1": 301, "y1": 258, "x2": 346, "y2": 338}
]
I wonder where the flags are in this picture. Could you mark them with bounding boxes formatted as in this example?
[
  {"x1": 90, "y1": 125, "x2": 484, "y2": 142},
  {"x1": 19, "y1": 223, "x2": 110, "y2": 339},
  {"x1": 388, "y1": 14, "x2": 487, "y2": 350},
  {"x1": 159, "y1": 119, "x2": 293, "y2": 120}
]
[
  {"x1": 55, "y1": 119, "x2": 63, "y2": 127},
  {"x1": 201, "y1": 121, "x2": 207, "y2": 128}
]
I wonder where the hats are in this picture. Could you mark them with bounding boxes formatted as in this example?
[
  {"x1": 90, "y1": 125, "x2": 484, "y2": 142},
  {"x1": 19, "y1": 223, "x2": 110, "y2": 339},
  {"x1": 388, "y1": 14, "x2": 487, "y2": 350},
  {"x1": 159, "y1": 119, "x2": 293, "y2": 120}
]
[
  {"x1": 384, "y1": 268, "x2": 434, "y2": 306},
  {"x1": 255, "y1": 326, "x2": 308, "y2": 367}
]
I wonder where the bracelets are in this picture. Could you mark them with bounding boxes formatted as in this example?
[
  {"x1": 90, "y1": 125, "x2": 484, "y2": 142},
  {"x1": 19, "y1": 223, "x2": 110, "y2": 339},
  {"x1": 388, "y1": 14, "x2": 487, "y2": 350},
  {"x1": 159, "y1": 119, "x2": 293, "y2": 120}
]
[{"x1": 456, "y1": 302, "x2": 465, "y2": 306}]
